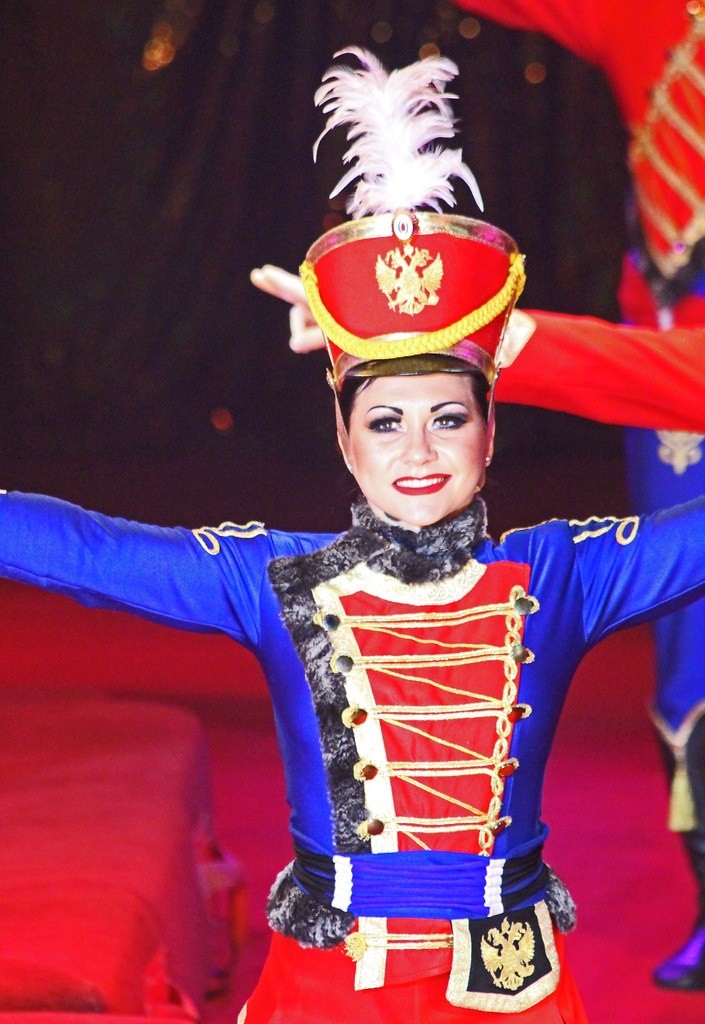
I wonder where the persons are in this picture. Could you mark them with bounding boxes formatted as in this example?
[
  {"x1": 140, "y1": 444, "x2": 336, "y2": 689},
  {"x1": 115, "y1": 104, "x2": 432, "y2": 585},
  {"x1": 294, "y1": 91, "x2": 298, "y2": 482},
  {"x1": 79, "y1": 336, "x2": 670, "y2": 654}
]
[{"x1": 0, "y1": 2, "x2": 705, "y2": 1024}]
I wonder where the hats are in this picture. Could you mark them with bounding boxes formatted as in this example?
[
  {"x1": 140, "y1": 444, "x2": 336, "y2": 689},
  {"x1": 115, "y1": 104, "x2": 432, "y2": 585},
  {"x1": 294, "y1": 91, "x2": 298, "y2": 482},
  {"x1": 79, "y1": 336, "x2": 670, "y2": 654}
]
[{"x1": 298, "y1": 46, "x2": 529, "y2": 394}]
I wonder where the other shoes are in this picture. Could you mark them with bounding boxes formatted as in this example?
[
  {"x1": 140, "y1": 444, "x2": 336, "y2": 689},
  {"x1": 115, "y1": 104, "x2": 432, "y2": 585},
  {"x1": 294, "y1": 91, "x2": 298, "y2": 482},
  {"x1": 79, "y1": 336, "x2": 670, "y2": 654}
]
[{"x1": 649, "y1": 923, "x2": 705, "y2": 989}]
